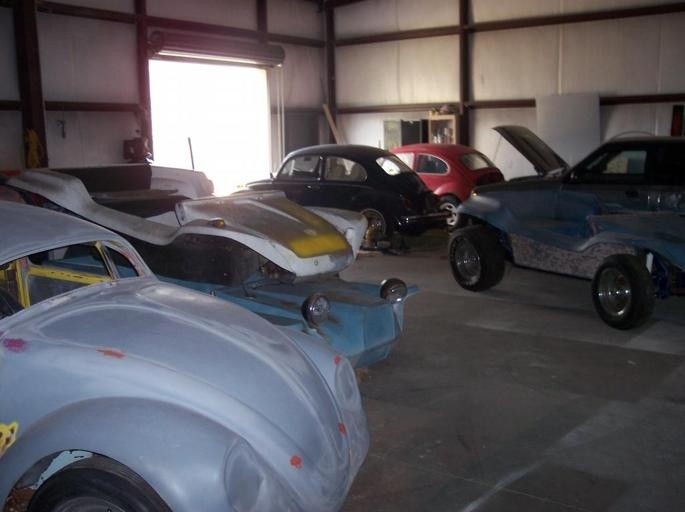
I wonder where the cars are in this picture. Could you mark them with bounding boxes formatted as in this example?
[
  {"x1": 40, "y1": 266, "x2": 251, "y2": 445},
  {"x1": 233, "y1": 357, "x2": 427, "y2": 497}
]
[{"x1": 0, "y1": 200, "x2": 372, "y2": 512}]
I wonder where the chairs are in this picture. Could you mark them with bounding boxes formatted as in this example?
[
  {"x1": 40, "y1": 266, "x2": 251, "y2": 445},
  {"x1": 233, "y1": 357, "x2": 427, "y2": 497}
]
[
  {"x1": 327, "y1": 163, "x2": 349, "y2": 181},
  {"x1": 418, "y1": 155, "x2": 473, "y2": 173},
  {"x1": 348, "y1": 163, "x2": 368, "y2": 181}
]
